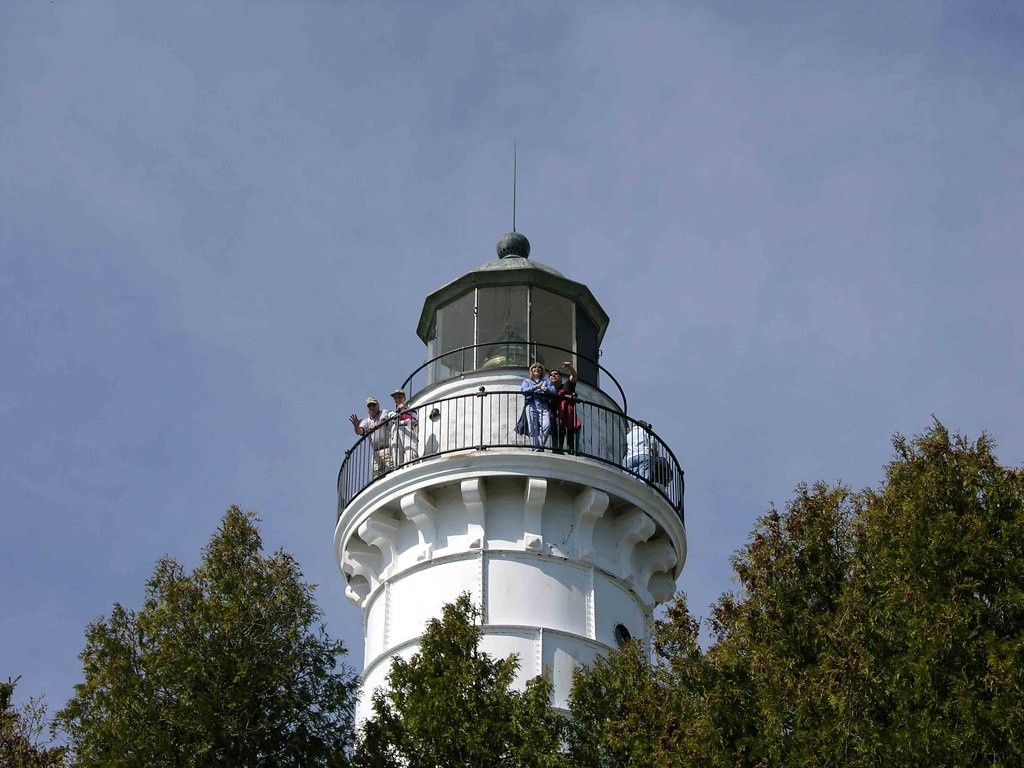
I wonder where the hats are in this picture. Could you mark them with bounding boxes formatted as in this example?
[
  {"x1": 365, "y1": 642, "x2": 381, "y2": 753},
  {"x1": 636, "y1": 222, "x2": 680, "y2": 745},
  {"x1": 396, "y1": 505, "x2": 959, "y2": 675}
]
[
  {"x1": 390, "y1": 389, "x2": 405, "y2": 397},
  {"x1": 366, "y1": 397, "x2": 378, "y2": 407}
]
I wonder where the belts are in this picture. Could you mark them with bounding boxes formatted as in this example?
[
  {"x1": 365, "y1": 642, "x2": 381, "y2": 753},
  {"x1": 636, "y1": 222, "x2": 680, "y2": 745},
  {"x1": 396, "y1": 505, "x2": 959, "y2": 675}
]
[
  {"x1": 399, "y1": 424, "x2": 406, "y2": 426},
  {"x1": 374, "y1": 445, "x2": 389, "y2": 451}
]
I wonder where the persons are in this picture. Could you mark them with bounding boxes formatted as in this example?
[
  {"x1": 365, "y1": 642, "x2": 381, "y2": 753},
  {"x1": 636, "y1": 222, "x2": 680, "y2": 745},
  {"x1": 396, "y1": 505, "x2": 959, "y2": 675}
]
[
  {"x1": 514, "y1": 361, "x2": 582, "y2": 455},
  {"x1": 388, "y1": 389, "x2": 420, "y2": 470},
  {"x1": 349, "y1": 397, "x2": 394, "y2": 480},
  {"x1": 623, "y1": 420, "x2": 672, "y2": 496}
]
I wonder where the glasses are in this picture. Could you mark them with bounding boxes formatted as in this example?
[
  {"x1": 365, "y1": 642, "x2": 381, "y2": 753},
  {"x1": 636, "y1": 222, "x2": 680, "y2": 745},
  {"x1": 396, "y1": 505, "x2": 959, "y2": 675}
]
[
  {"x1": 368, "y1": 403, "x2": 376, "y2": 407},
  {"x1": 394, "y1": 396, "x2": 401, "y2": 399}
]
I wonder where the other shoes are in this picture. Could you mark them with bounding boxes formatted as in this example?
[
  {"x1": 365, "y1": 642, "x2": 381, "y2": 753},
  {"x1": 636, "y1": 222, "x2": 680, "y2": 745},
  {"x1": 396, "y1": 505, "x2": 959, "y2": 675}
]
[{"x1": 532, "y1": 449, "x2": 543, "y2": 452}]
